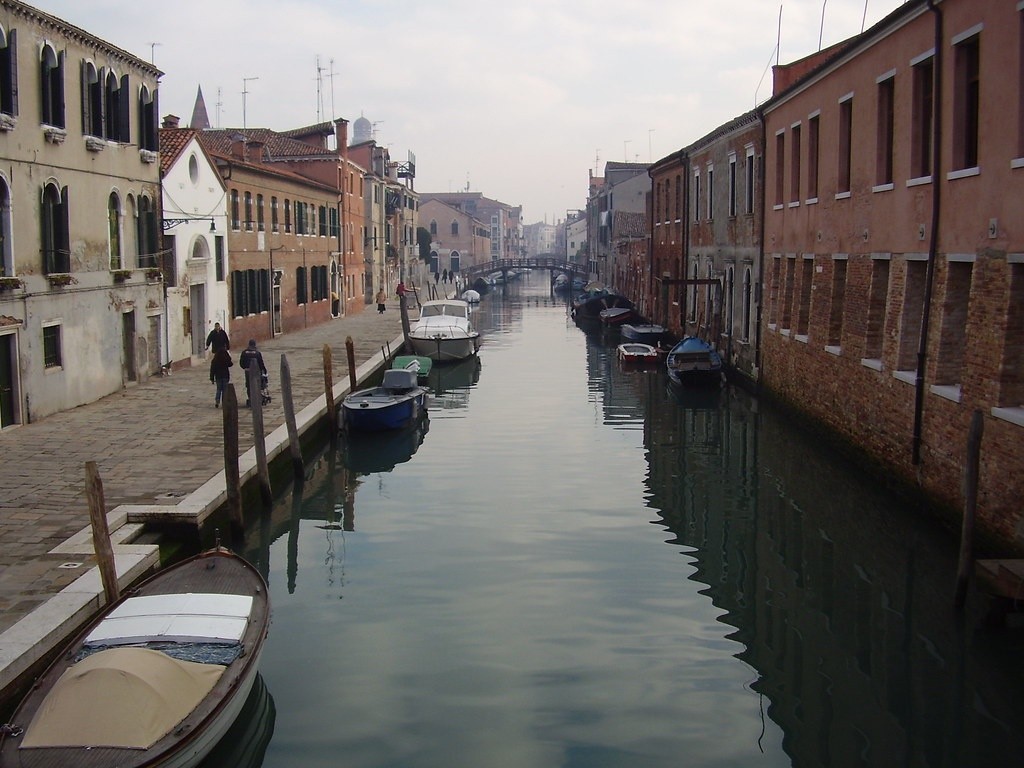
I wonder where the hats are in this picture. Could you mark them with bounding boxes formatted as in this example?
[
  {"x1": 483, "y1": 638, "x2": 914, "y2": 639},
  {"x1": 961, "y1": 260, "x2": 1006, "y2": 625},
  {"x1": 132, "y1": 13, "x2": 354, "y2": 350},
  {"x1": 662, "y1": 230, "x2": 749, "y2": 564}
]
[{"x1": 249, "y1": 340, "x2": 255, "y2": 345}]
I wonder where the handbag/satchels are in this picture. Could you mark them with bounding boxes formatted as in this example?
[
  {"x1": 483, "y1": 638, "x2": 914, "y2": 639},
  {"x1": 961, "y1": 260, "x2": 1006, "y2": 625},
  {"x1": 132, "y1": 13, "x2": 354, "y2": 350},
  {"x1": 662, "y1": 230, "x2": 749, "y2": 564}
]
[
  {"x1": 396, "y1": 284, "x2": 401, "y2": 294},
  {"x1": 376, "y1": 293, "x2": 380, "y2": 303}
]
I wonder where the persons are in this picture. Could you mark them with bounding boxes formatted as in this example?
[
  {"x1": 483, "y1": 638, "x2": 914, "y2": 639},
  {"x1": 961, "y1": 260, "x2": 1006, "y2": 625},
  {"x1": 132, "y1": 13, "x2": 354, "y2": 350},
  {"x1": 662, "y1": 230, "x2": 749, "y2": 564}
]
[
  {"x1": 205, "y1": 322, "x2": 230, "y2": 353},
  {"x1": 449, "y1": 270, "x2": 454, "y2": 282},
  {"x1": 434, "y1": 271, "x2": 439, "y2": 285},
  {"x1": 332, "y1": 291, "x2": 339, "y2": 318},
  {"x1": 442, "y1": 268, "x2": 447, "y2": 284},
  {"x1": 210, "y1": 347, "x2": 234, "y2": 408},
  {"x1": 240, "y1": 339, "x2": 266, "y2": 406},
  {"x1": 376, "y1": 288, "x2": 387, "y2": 314},
  {"x1": 396, "y1": 282, "x2": 408, "y2": 299}
]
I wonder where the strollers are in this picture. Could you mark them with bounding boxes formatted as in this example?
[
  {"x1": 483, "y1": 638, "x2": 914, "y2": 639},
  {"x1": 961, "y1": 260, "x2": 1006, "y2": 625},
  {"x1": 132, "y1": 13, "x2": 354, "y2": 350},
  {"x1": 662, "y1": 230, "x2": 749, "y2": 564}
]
[{"x1": 246, "y1": 369, "x2": 272, "y2": 405}]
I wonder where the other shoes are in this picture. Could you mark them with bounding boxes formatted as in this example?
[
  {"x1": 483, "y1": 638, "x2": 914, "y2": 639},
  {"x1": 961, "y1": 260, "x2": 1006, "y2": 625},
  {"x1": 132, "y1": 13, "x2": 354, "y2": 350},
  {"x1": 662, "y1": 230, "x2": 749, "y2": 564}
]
[{"x1": 215, "y1": 403, "x2": 219, "y2": 408}]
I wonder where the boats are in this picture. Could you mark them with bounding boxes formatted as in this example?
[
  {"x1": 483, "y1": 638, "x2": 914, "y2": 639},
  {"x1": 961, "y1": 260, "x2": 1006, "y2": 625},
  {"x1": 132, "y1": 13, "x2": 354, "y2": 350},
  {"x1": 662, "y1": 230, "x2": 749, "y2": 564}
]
[
  {"x1": 620, "y1": 323, "x2": 665, "y2": 343},
  {"x1": 341, "y1": 358, "x2": 432, "y2": 431},
  {"x1": 461, "y1": 289, "x2": 480, "y2": 303},
  {"x1": 0, "y1": 526, "x2": 272, "y2": 767},
  {"x1": 392, "y1": 352, "x2": 433, "y2": 377},
  {"x1": 600, "y1": 307, "x2": 632, "y2": 325},
  {"x1": 408, "y1": 298, "x2": 481, "y2": 360},
  {"x1": 616, "y1": 343, "x2": 659, "y2": 362},
  {"x1": 666, "y1": 336, "x2": 722, "y2": 382},
  {"x1": 473, "y1": 267, "x2": 586, "y2": 294}
]
